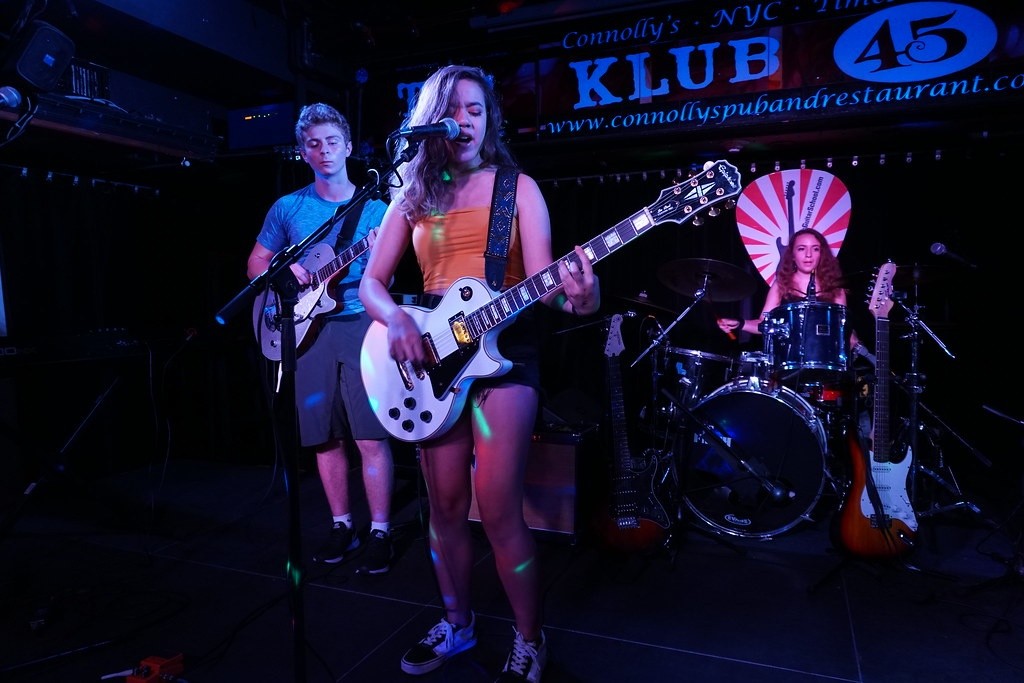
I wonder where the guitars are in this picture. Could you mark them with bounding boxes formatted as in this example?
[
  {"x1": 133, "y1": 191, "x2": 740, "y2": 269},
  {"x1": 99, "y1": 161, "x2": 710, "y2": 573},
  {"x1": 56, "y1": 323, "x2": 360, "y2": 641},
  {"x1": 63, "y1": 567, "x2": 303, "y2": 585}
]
[
  {"x1": 253, "y1": 224, "x2": 382, "y2": 361},
  {"x1": 836, "y1": 260, "x2": 923, "y2": 555},
  {"x1": 358, "y1": 155, "x2": 749, "y2": 443},
  {"x1": 595, "y1": 311, "x2": 677, "y2": 555}
]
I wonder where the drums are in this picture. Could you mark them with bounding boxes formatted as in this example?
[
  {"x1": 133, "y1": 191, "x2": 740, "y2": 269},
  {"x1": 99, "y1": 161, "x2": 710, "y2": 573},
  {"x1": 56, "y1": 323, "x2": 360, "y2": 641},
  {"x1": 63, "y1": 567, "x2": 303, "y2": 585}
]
[
  {"x1": 734, "y1": 299, "x2": 849, "y2": 382},
  {"x1": 659, "y1": 345, "x2": 736, "y2": 414},
  {"x1": 671, "y1": 376, "x2": 830, "y2": 539}
]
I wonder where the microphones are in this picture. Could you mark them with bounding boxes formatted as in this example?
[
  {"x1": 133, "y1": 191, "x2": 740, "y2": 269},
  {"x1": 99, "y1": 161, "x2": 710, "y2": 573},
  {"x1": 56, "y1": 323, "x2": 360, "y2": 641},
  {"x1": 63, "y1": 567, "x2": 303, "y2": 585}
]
[
  {"x1": 655, "y1": 320, "x2": 671, "y2": 345},
  {"x1": 930, "y1": 242, "x2": 978, "y2": 270},
  {"x1": 389, "y1": 117, "x2": 460, "y2": 140},
  {"x1": 763, "y1": 481, "x2": 785, "y2": 502},
  {"x1": 807, "y1": 271, "x2": 816, "y2": 300}
]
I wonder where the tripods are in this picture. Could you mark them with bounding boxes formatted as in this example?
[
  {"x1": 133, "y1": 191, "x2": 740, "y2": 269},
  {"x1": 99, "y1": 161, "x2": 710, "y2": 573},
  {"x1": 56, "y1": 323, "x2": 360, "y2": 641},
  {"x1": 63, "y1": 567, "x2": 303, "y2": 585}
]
[{"x1": 883, "y1": 277, "x2": 1018, "y2": 544}]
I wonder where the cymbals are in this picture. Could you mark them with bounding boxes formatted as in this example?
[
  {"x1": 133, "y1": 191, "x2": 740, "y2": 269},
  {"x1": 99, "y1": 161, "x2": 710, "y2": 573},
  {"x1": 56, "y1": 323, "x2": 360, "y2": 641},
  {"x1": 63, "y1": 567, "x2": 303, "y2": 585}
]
[
  {"x1": 623, "y1": 291, "x2": 674, "y2": 315},
  {"x1": 667, "y1": 256, "x2": 751, "y2": 304}
]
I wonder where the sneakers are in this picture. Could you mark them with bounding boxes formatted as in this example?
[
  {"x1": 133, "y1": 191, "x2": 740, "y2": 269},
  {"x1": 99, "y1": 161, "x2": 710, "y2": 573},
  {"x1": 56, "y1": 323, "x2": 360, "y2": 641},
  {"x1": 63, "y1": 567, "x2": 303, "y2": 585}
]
[
  {"x1": 313, "y1": 521, "x2": 359, "y2": 563},
  {"x1": 400, "y1": 611, "x2": 477, "y2": 675},
  {"x1": 354, "y1": 530, "x2": 395, "y2": 576},
  {"x1": 494, "y1": 626, "x2": 547, "y2": 683}
]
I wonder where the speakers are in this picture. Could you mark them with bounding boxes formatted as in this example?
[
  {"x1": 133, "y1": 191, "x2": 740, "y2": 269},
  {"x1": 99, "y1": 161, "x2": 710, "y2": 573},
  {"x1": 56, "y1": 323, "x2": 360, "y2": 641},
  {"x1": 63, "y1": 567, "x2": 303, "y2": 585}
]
[
  {"x1": 465, "y1": 421, "x2": 601, "y2": 537},
  {"x1": 16, "y1": 333, "x2": 154, "y2": 484},
  {"x1": 3, "y1": 19, "x2": 75, "y2": 93}
]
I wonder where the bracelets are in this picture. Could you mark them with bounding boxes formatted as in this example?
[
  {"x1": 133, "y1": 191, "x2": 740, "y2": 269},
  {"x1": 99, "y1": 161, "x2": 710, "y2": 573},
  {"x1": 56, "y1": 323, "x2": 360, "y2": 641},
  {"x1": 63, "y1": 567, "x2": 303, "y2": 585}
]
[
  {"x1": 736, "y1": 319, "x2": 745, "y2": 329},
  {"x1": 551, "y1": 292, "x2": 574, "y2": 315}
]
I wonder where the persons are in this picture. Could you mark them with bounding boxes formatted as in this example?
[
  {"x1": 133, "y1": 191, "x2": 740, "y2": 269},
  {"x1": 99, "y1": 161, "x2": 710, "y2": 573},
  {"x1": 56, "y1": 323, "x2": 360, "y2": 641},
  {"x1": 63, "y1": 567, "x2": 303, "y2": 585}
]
[
  {"x1": 359, "y1": 67, "x2": 599, "y2": 683},
  {"x1": 246, "y1": 103, "x2": 394, "y2": 575},
  {"x1": 716, "y1": 229, "x2": 858, "y2": 352}
]
